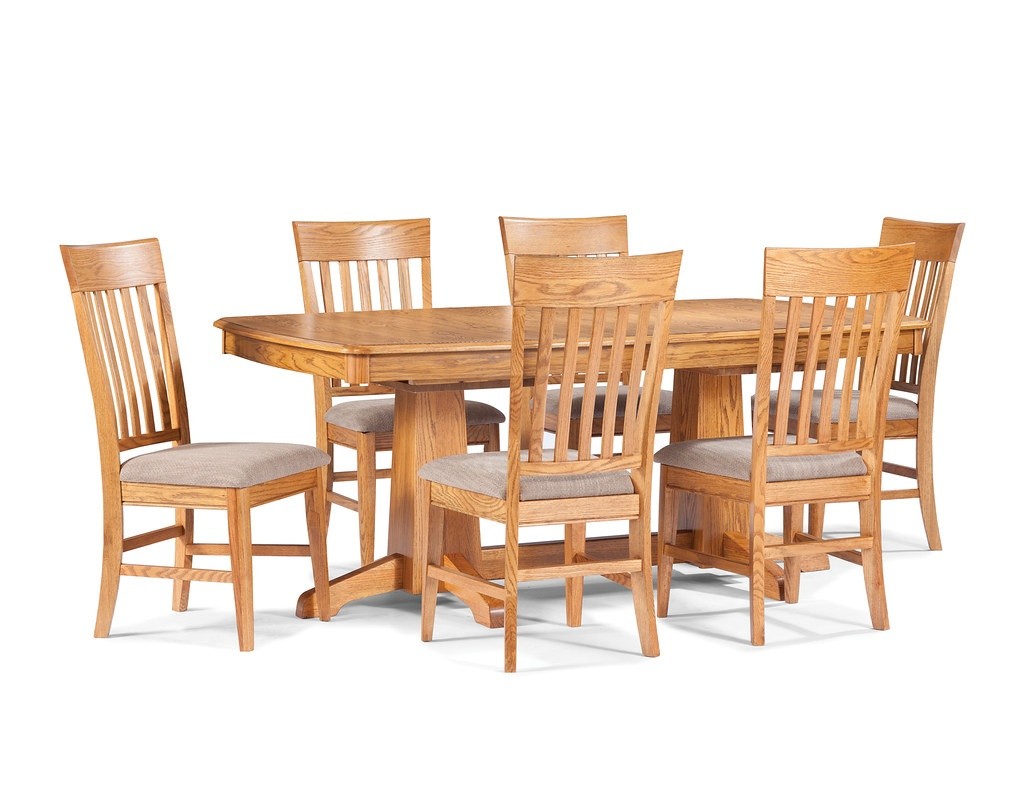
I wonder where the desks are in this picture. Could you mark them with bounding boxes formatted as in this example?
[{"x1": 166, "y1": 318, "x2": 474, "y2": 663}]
[{"x1": 214, "y1": 299, "x2": 930, "y2": 629}]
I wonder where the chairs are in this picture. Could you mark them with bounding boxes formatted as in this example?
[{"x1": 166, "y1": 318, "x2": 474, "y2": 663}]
[
  {"x1": 60, "y1": 237, "x2": 333, "y2": 650},
  {"x1": 652, "y1": 242, "x2": 917, "y2": 645},
  {"x1": 500, "y1": 215, "x2": 675, "y2": 454},
  {"x1": 753, "y1": 216, "x2": 966, "y2": 550},
  {"x1": 291, "y1": 217, "x2": 505, "y2": 567},
  {"x1": 416, "y1": 250, "x2": 688, "y2": 674}
]
